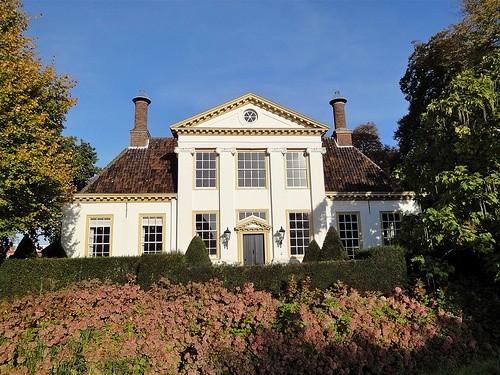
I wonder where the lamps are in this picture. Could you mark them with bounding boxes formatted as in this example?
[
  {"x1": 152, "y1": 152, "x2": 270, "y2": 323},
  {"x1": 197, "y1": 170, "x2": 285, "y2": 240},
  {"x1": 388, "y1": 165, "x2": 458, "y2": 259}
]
[
  {"x1": 223, "y1": 227, "x2": 231, "y2": 249},
  {"x1": 278, "y1": 226, "x2": 286, "y2": 248}
]
